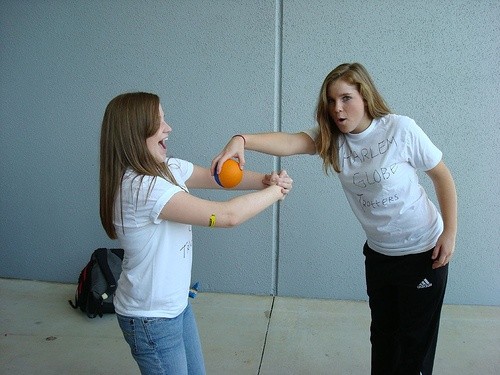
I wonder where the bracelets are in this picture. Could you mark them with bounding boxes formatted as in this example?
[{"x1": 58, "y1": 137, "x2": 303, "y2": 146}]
[{"x1": 232, "y1": 134, "x2": 246, "y2": 146}]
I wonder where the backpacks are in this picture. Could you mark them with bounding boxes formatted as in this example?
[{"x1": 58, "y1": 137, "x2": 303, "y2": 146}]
[{"x1": 68, "y1": 248, "x2": 125, "y2": 318}]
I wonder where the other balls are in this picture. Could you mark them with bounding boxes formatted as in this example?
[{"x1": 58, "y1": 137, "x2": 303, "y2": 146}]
[{"x1": 213, "y1": 159, "x2": 244, "y2": 189}]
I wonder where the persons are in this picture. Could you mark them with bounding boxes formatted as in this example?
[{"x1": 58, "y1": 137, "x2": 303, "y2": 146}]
[
  {"x1": 100, "y1": 92, "x2": 293, "y2": 375},
  {"x1": 210, "y1": 62, "x2": 458, "y2": 375}
]
